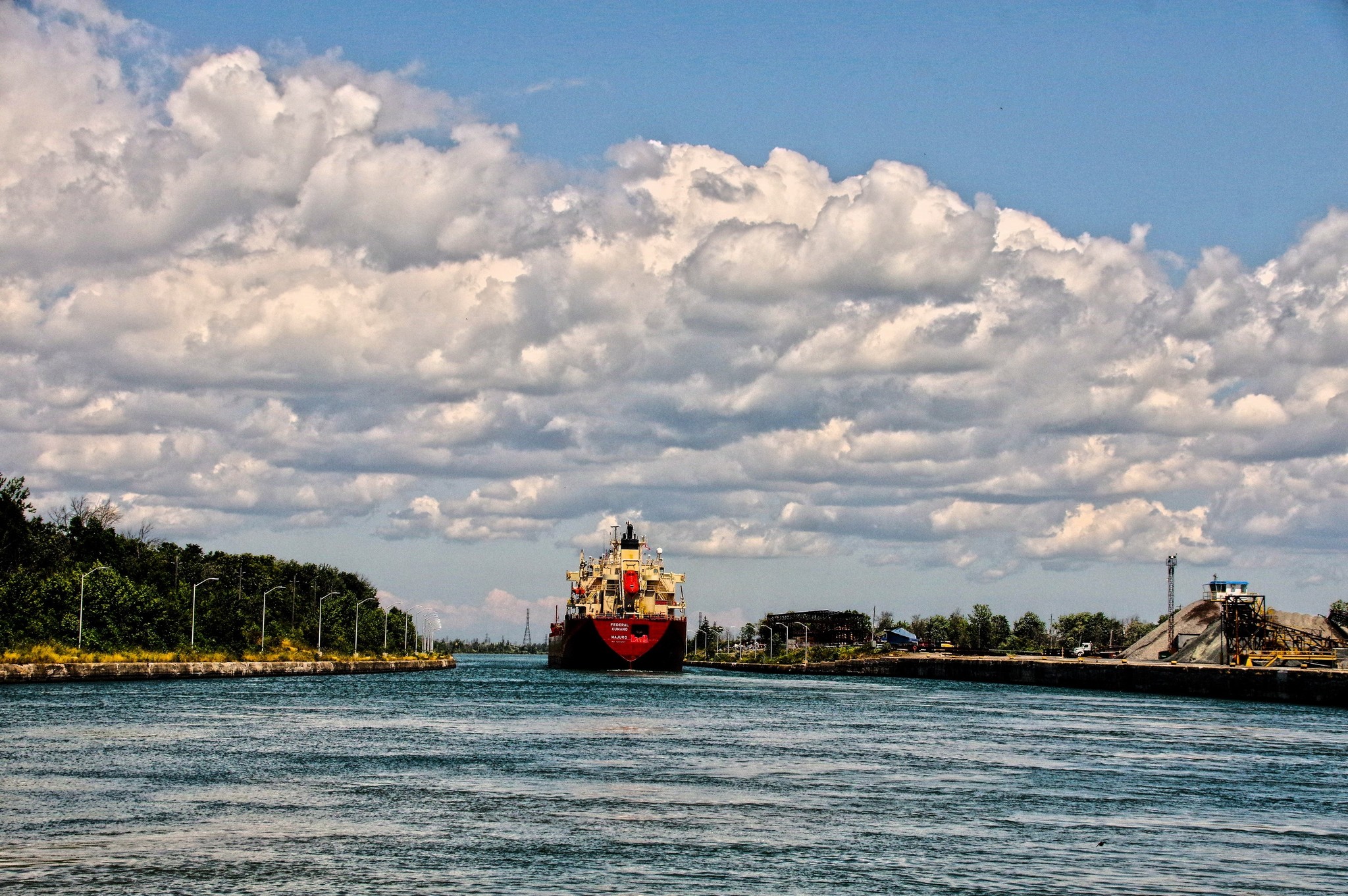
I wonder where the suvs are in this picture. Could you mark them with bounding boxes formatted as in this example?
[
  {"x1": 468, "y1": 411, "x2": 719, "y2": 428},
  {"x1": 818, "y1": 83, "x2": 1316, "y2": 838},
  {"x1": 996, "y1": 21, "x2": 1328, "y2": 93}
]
[{"x1": 876, "y1": 644, "x2": 884, "y2": 648}]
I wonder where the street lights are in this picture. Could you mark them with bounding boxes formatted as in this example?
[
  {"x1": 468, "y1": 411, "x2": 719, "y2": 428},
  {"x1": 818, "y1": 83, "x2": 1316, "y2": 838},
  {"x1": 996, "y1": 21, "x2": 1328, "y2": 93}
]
[
  {"x1": 384, "y1": 602, "x2": 404, "y2": 649},
  {"x1": 709, "y1": 629, "x2": 719, "y2": 651},
  {"x1": 731, "y1": 626, "x2": 741, "y2": 658},
  {"x1": 404, "y1": 605, "x2": 442, "y2": 654},
  {"x1": 795, "y1": 622, "x2": 807, "y2": 662},
  {"x1": 192, "y1": 578, "x2": 220, "y2": 651},
  {"x1": 760, "y1": 624, "x2": 772, "y2": 661},
  {"x1": 355, "y1": 598, "x2": 377, "y2": 652},
  {"x1": 687, "y1": 630, "x2": 697, "y2": 655},
  {"x1": 318, "y1": 592, "x2": 341, "y2": 652},
  {"x1": 775, "y1": 622, "x2": 788, "y2": 657},
  {"x1": 697, "y1": 629, "x2": 706, "y2": 657},
  {"x1": 745, "y1": 625, "x2": 756, "y2": 659},
  {"x1": 719, "y1": 628, "x2": 729, "y2": 655},
  {"x1": 78, "y1": 567, "x2": 110, "y2": 650},
  {"x1": 261, "y1": 586, "x2": 286, "y2": 651}
]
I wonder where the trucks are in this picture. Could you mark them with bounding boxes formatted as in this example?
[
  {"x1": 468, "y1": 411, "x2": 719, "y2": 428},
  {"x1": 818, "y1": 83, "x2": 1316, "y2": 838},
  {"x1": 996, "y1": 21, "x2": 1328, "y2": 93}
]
[{"x1": 1074, "y1": 643, "x2": 1116, "y2": 658}]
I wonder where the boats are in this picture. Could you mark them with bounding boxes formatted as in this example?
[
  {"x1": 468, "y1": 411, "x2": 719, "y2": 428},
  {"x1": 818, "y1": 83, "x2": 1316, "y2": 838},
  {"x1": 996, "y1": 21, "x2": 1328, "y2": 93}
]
[
  {"x1": 572, "y1": 587, "x2": 585, "y2": 594},
  {"x1": 624, "y1": 571, "x2": 639, "y2": 594}
]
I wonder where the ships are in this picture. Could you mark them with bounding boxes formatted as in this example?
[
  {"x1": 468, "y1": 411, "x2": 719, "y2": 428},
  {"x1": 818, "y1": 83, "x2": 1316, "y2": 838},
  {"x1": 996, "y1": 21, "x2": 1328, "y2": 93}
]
[{"x1": 547, "y1": 519, "x2": 688, "y2": 675}]
